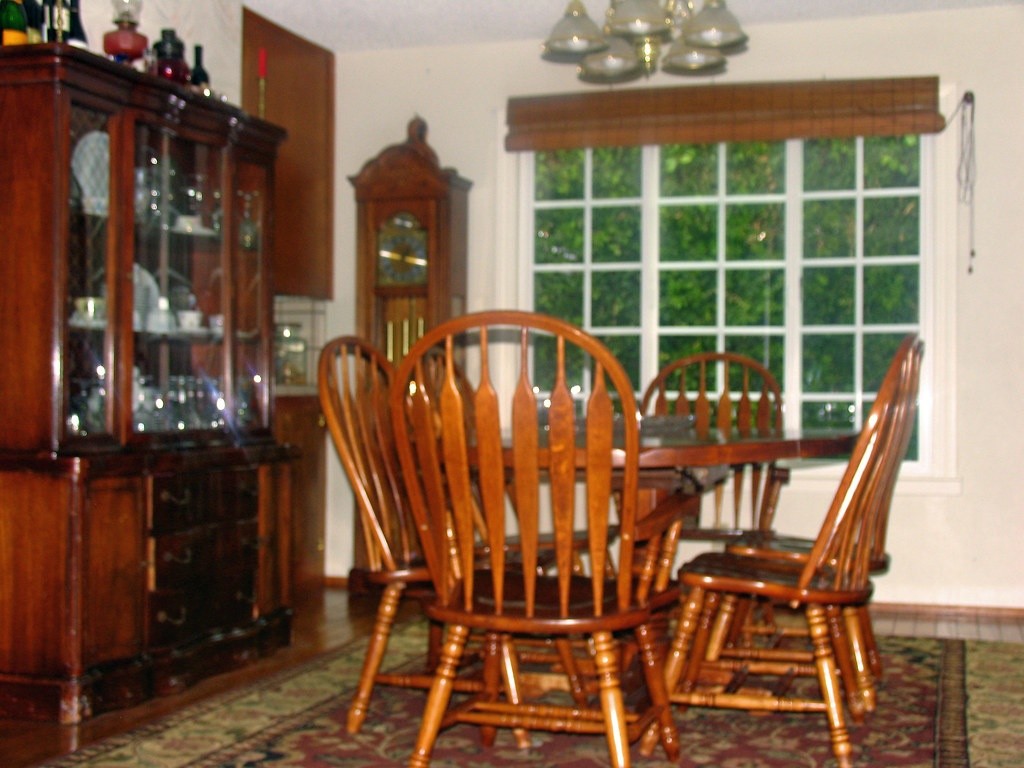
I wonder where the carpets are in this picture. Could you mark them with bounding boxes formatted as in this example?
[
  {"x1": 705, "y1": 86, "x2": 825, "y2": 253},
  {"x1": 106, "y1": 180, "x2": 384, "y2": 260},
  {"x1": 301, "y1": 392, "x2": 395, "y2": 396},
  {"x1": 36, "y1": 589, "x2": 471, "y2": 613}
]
[{"x1": 184, "y1": 636, "x2": 969, "y2": 768}]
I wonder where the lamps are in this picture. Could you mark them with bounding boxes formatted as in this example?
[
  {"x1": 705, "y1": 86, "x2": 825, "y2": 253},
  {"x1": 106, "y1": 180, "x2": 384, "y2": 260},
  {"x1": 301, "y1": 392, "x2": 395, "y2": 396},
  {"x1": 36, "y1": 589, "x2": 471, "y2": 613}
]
[{"x1": 543, "y1": 0, "x2": 747, "y2": 85}]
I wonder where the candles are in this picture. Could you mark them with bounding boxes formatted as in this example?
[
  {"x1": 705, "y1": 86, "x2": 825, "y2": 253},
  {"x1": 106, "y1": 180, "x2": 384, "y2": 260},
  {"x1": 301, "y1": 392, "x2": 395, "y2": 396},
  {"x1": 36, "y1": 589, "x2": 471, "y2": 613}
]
[{"x1": 257, "y1": 46, "x2": 269, "y2": 80}]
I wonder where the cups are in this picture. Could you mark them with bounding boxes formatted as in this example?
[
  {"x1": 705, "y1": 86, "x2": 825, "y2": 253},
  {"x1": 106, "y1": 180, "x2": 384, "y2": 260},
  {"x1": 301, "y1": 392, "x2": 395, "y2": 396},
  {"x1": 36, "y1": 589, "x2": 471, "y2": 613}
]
[
  {"x1": 207, "y1": 314, "x2": 225, "y2": 330},
  {"x1": 73, "y1": 297, "x2": 108, "y2": 321},
  {"x1": 176, "y1": 309, "x2": 203, "y2": 329}
]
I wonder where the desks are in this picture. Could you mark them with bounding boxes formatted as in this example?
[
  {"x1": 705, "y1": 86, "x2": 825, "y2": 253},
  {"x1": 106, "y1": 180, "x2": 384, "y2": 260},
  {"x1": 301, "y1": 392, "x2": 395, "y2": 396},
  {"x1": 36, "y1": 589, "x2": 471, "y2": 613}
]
[{"x1": 418, "y1": 416, "x2": 862, "y2": 573}]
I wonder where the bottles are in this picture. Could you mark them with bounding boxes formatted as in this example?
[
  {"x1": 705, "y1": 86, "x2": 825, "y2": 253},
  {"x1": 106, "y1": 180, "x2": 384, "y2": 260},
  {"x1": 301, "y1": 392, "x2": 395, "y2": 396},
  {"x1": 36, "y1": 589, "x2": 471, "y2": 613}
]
[
  {"x1": 237, "y1": 192, "x2": 257, "y2": 249},
  {"x1": 188, "y1": 46, "x2": 210, "y2": 97},
  {"x1": 0, "y1": 0, "x2": 87, "y2": 46},
  {"x1": 148, "y1": 29, "x2": 191, "y2": 83}
]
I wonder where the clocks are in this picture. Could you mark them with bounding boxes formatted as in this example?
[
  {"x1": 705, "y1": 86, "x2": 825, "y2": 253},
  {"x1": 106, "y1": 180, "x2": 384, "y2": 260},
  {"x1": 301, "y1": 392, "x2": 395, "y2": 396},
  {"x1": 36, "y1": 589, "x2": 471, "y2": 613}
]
[{"x1": 350, "y1": 116, "x2": 471, "y2": 600}]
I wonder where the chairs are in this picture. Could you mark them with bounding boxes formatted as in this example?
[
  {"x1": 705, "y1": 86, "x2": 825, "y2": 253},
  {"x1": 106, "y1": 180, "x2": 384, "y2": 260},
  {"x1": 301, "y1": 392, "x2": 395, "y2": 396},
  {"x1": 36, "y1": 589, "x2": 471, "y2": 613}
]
[
  {"x1": 319, "y1": 335, "x2": 591, "y2": 752},
  {"x1": 703, "y1": 338, "x2": 928, "y2": 712},
  {"x1": 407, "y1": 353, "x2": 617, "y2": 580},
  {"x1": 641, "y1": 333, "x2": 923, "y2": 768},
  {"x1": 390, "y1": 311, "x2": 683, "y2": 768},
  {"x1": 639, "y1": 353, "x2": 790, "y2": 543}
]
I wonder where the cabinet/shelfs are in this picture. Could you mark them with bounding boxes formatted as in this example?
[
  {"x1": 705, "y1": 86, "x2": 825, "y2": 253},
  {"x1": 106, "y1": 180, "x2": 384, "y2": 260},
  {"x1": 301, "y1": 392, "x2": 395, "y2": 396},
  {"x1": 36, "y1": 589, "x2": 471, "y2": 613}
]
[{"x1": 0, "y1": 46, "x2": 300, "y2": 716}]
